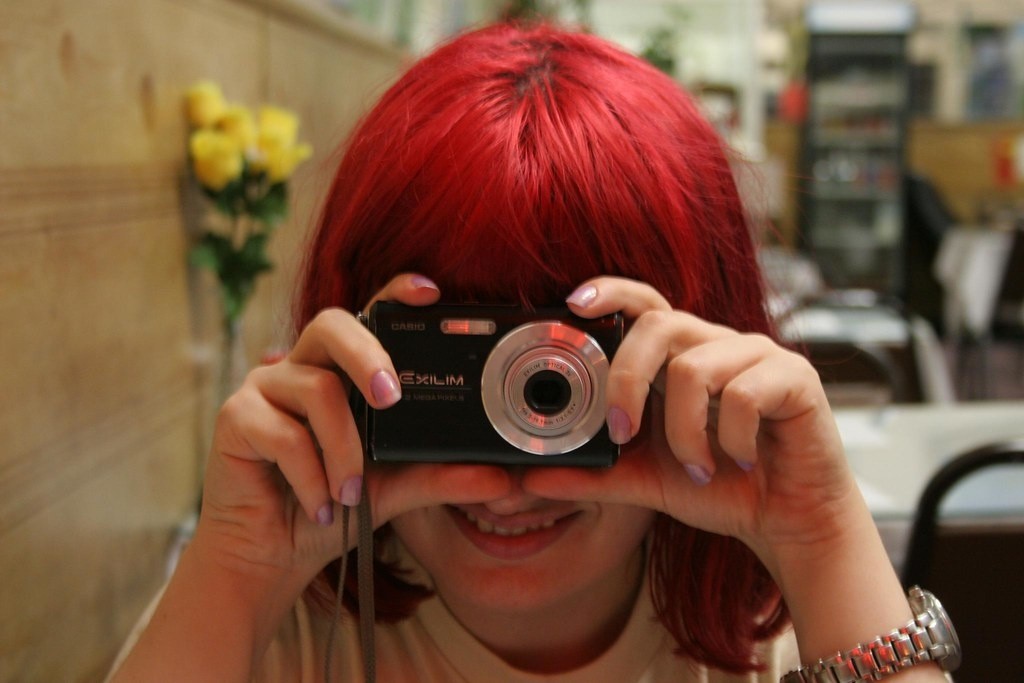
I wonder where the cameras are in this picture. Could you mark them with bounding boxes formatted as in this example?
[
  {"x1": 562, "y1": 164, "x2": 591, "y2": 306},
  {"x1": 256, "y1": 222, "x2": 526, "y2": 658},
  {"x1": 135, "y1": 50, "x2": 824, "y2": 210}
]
[{"x1": 355, "y1": 298, "x2": 629, "y2": 469}]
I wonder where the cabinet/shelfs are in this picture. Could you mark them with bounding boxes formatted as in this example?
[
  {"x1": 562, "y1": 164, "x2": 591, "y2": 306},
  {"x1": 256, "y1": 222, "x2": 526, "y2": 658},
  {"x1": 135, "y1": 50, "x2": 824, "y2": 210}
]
[{"x1": 794, "y1": 28, "x2": 911, "y2": 313}]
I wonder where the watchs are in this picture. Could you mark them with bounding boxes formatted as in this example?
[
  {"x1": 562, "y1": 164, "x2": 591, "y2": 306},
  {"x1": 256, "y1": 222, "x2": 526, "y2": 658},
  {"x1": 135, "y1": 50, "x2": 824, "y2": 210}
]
[{"x1": 778, "y1": 583, "x2": 961, "y2": 683}]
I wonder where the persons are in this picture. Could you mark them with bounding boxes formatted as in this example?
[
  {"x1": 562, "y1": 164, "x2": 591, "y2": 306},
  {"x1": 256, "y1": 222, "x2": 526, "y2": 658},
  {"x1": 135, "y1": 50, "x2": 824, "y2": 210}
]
[{"x1": 101, "y1": 21, "x2": 969, "y2": 683}]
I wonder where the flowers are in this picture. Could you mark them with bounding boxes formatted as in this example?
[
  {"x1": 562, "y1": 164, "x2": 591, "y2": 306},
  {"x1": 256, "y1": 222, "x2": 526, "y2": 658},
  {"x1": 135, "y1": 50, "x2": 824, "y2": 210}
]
[{"x1": 184, "y1": 76, "x2": 314, "y2": 412}]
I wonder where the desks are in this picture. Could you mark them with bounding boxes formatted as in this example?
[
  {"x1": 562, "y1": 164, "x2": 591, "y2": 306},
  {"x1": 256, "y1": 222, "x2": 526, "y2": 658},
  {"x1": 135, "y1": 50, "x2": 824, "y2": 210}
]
[
  {"x1": 778, "y1": 303, "x2": 909, "y2": 405},
  {"x1": 833, "y1": 403, "x2": 1024, "y2": 568}
]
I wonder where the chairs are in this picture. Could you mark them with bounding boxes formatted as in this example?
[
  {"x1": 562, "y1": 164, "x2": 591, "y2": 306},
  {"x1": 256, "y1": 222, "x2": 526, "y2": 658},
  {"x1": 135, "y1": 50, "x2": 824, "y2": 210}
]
[
  {"x1": 899, "y1": 435, "x2": 1024, "y2": 683},
  {"x1": 793, "y1": 339, "x2": 904, "y2": 406}
]
